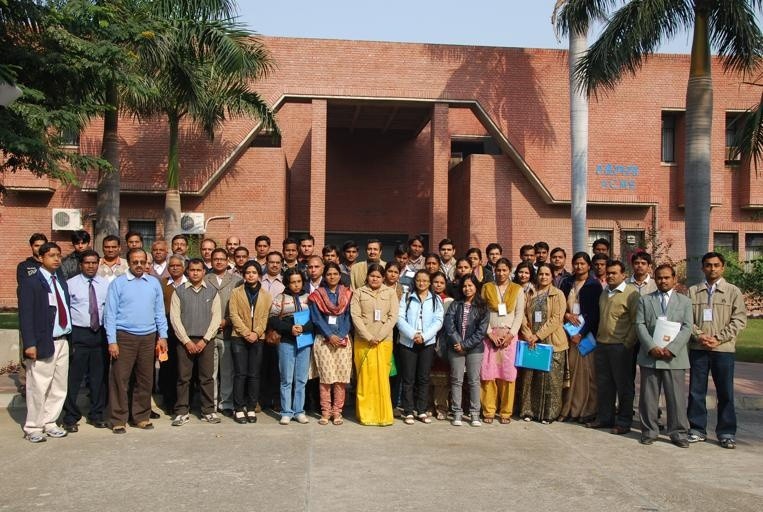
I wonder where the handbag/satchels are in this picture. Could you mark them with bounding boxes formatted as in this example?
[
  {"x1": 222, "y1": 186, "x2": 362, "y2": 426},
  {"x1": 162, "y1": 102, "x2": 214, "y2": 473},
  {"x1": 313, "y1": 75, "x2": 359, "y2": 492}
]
[
  {"x1": 263, "y1": 292, "x2": 285, "y2": 344},
  {"x1": 435, "y1": 329, "x2": 447, "y2": 361}
]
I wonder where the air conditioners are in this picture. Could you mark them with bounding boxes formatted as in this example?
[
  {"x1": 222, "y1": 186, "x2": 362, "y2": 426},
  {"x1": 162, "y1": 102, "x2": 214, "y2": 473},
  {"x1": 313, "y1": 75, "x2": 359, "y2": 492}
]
[
  {"x1": 180, "y1": 213, "x2": 205, "y2": 235},
  {"x1": 52, "y1": 208, "x2": 82, "y2": 233}
]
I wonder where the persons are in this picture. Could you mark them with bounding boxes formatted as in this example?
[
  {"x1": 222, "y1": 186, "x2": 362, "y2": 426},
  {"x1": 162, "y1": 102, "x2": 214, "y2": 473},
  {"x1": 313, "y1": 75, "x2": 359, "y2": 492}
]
[
  {"x1": 16, "y1": 234, "x2": 48, "y2": 396},
  {"x1": 63, "y1": 251, "x2": 110, "y2": 433},
  {"x1": 444, "y1": 275, "x2": 491, "y2": 426},
  {"x1": 550, "y1": 248, "x2": 571, "y2": 290},
  {"x1": 148, "y1": 240, "x2": 172, "y2": 283},
  {"x1": 426, "y1": 272, "x2": 450, "y2": 420},
  {"x1": 451, "y1": 258, "x2": 473, "y2": 295},
  {"x1": 303, "y1": 256, "x2": 325, "y2": 294},
  {"x1": 635, "y1": 264, "x2": 693, "y2": 449},
  {"x1": 533, "y1": 243, "x2": 550, "y2": 274},
  {"x1": 266, "y1": 269, "x2": 315, "y2": 425},
  {"x1": 593, "y1": 238, "x2": 610, "y2": 257},
  {"x1": 424, "y1": 253, "x2": 440, "y2": 274},
  {"x1": 61, "y1": 230, "x2": 90, "y2": 282},
  {"x1": 309, "y1": 262, "x2": 353, "y2": 426},
  {"x1": 96, "y1": 235, "x2": 130, "y2": 282},
  {"x1": 202, "y1": 248, "x2": 244, "y2": 417},
  {"x1": 170, "y1": 259, "x2": 221, "y2": 425},
  {"x1": 323, "y1": 246, "x2": 351, "y2": 289},
  {"x1": 513, "y1": 261, "x2": 537, "y2": 298},
  {"x1": 486, "y1": 243, "x2": 503, "y2": 284},
  {"x1": 125, "y1": 230, "x2": 144, "y2": 249},
  {"x1": 586, "y1": 261, "x2": 641, "y2": 434},
  {"x1": 589, "y1": 253, "x2": 612, "y2": 290},
  {"x1": 228, "y1": 260, "x2": 272, "y2": 424},
  {"x1": 172, "y1": 236, "x2": 190, "y2": 260},
  {"x1": 481, "y1": 258, "x2": 524, "y2": 425},
  {"x1": 146, "y1": 251, "x2": 162, "y2": 418},
  {"x1": 466, "y1": 248, "x2": 494, "y2": 294},
  {"x1": 396, "y1": 269, "x2": 443, "y2": 426},
  {"x1": 395, "y1": 246, "x2": 415, "y2": 279},
  {"x1": 185, "y1": 238, "x2": 213, "y2": 280},
  {"x1": 300, "y1": 233, "x2": 315, "y2": 268},
  {"x1": 160, "y1": 255, "x2": 192, "y2": 414},
  {"x1": 383, "y1": 263, "x2": 404, "y2": 417},
  {"x1": 687, "y1": 252, "x2": 747, "y2": 449},
  {"x1": 519, "y1": 264, "x2": 570, "y2": 425},
  {"x1": 339, "y1": 242, "x2": 360, "y2": 276},
  {"x1": 103, "y1": 249, "x2": 168, "y2": 435},
  {"x1": 624, "y1": 252, "x2": 665, "y2": 430},
  {"x1": 280, "y1": 239, "x2": 309, "y2": 277},
  {"x1": 520, "y1": 245, "x2": 539, "y2": 275},
  {"x1": 406, "y1": 234, "x2": 426, "y2": 274},
  {"x1": 18, "y1": 243, "x2": 72, "y2": 442},
  {"x1": 257, "y1": 252, "x2": 285, "y2": 411},
  {"x1": 351, "y1": 238, "x2": 387, "y2": 291},
  {"x1": 228, "y1": 247, "x2": 249, "y2": 279},
  {"x1": 248, "y1": 236, "x2": 271, "y2": 275},
  {"x1": 559, "y1": 252, "x2": 603, "y2": 423},
  {"x1": 439, "y1": 239, "x2": 458, "y2": 282},
  {"x1": 351, "y1": 264, "x2": 398, "y2": 426},
  {"x1": 226, "y1": 236, "x2": 241, "y2": 270}
]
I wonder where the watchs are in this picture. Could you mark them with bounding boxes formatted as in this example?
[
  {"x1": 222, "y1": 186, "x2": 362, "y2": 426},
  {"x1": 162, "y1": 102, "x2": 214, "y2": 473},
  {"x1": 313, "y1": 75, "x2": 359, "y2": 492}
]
[{"x1": 203, "y1": 338, "x2": 208, "y2": 344}]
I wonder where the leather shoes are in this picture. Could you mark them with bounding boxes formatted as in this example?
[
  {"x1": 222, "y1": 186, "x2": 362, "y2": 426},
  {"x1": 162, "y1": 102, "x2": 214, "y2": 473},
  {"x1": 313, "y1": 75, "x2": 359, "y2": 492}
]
[
  {"x1": 235, "y1": 412, "x2": 246, "y2": 423},
  {"x1": 542, "y1": 420, "x2": 550, "y2": 424},
  {"x1": 150, "y1": 411, "x2": 160, "y2": 418},
  {"x1": 483, "y1": 416, "x2": 492, "y2": 423},
  {"x1": 64, "y1": 424, "x2": 79, "y2": 432},
  {"x1": 674, "y1": 439, "x2": 688, "y2": 448},
  {"x1": 95, "y1": 422, "x2": 108, "y2": 427},
  {"x1": 523, "y1": 416, "x2": 532, "y2": 422},
  {"x1": 611, "y1": 426, "x2": 629, "y2": 434},
  {"x1": 641, "y1": 437, "x2": 653, "y2": 445},
  {"x1": 247, "y1": 413, "x2": 257, "y2": 423},
  {"x1": 587, "y1": 420, "x2": 606, "y2": 428},
  {"x1": 223, "y1": 408, "x2": 233, "y2": 416},
  {"x1": 501, "y1": 417, "x2": 510, "y2": 424},
  {"x1": 658, "y1": 420, "x2": 664, "y2": 429}
]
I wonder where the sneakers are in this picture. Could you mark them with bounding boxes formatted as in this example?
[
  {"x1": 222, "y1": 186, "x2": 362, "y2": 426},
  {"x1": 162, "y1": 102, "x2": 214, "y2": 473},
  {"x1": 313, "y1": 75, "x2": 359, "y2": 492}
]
[
  {"x1": 319, "y1": 418, "x2": 328, "y2": 425},
  {"x1": 471, "y1": 415, "x2": 480, "y2": 426},
  {"x1": 417, "y1": 414, "x2": 431, "y2": 424},
  {"x1": 200, "y1": 412, "x2": 221, "y2": 424},
  {"x1": 43, "y1": 423, "x2": 67, "y2": 437},
  {"x1": 279, "y1": 416, "x2": 290, "y2": 424},
  {"x1": 720, "y1": 438, "x2": 736, "y2": 449},
  {"x1": 687, "y1": 432, "x2": 705, "y2": 442},
  {"x1": 333, "y1": 417, "x2": 343, "y2": 425},
  {"x1": 25, "y1": 430, "x2": 48, "y2": 442},
  {"x1": 137, "y1": 421, "x2": 153, "y2": 429},
  {"x1": 298, "y1": 415, "x2": 309, "y2": 423},
  {"x1": 437, "y1": 410, "x2": 446, "y2": 419},
  {"x1": 393, "y1": 408, "x2": 401, "y2": 418},
  {"x1": 404, "y1": 413, "x2": 414, "y2": 425},
  {"x1": 171, "y1": 413, "x2": 189, "y2": 425},
  {"x1": 451, "y1": 415, "x2": 461, "y2": 426},
  {"x1": 112, "y1": 426, "x2": 127, "y2": 433}
]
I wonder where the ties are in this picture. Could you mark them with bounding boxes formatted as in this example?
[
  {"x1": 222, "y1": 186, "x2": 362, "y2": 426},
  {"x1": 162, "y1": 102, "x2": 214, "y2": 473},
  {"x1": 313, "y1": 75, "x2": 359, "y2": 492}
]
[
  {"x1": 52, "y1": 276, "x2": 69, "y2": 329},
  {"x1": 660, "y1": 292, "x2": 666, "y2": 315},
  {"x1": 708, "y1": 286, "x2": 712, "y2": 309},
  {"x1": 89, "y1": 279, "x2": 99, "y2": 332}
]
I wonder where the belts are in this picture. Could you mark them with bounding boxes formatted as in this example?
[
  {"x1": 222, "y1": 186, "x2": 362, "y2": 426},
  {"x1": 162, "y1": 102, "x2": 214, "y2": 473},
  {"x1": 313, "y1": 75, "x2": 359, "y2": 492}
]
[{"x1": 52, "y1": 335, "x2": 64, "y2": 340}]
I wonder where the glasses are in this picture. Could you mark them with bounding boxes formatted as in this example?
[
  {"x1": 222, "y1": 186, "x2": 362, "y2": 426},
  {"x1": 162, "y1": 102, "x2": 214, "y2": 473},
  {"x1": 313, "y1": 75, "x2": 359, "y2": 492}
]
[
  {"x1": 44, "y1": 254, "x2": 61, "y2": 258},
  {"x1": 131, "y1": 260, "x2": 146, "y2": 264}
]
